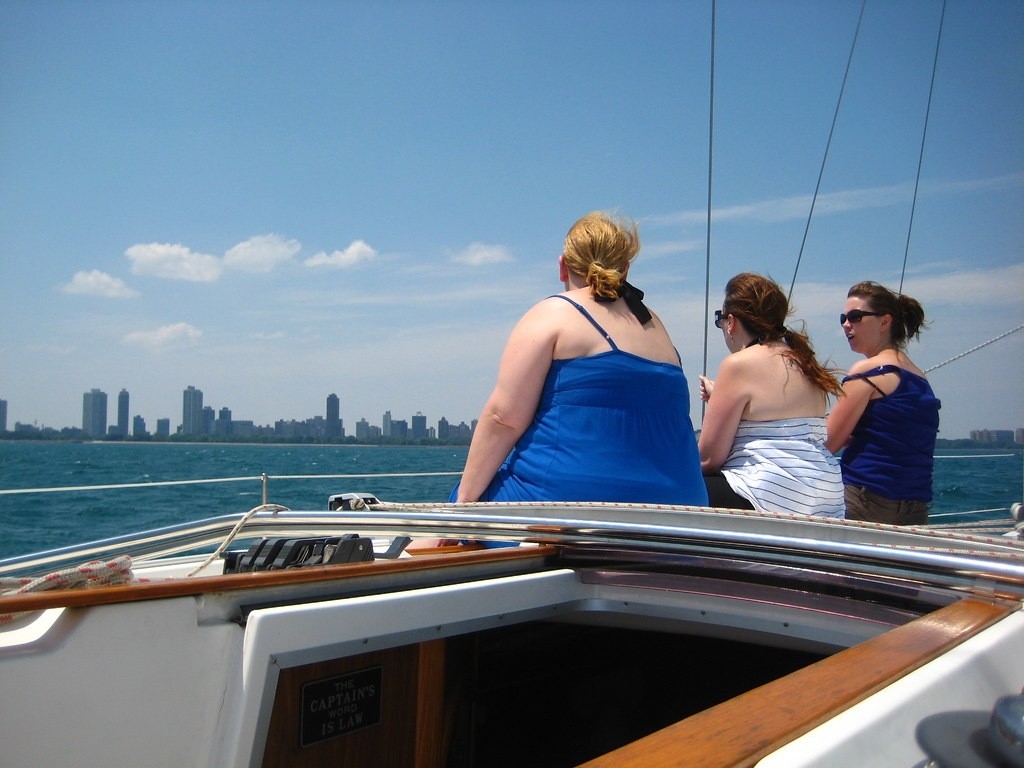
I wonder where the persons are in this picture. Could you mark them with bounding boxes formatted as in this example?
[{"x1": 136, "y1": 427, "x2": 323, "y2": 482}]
[
  {"x1": 435, "y1": 211, "x2": 709, "y2": 549},
  {"x1": 822, "y1": 281, "x2": 941, "y2": 526},
  {"x1": 697, "y1": 272, "x2": 846, "y2": 519}
]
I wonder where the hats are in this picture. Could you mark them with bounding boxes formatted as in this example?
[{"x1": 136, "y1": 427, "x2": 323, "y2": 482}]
[{"x1": 915, "y1": 694, "x2": 1024, "y2": 768}]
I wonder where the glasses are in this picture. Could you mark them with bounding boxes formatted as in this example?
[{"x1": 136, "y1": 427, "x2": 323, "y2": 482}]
[
  {"x1": 715, "y1": 310, "x2": 728, "y2": 328},
  {"x1": 840, "y1": 309, "x2": 884, "y2": 325}
]
